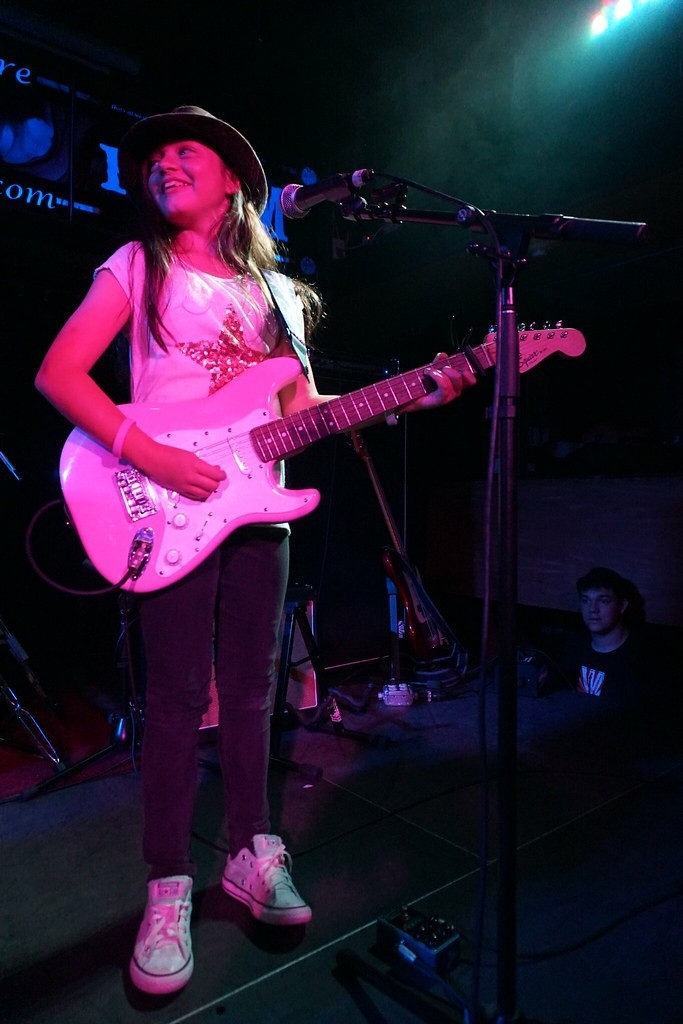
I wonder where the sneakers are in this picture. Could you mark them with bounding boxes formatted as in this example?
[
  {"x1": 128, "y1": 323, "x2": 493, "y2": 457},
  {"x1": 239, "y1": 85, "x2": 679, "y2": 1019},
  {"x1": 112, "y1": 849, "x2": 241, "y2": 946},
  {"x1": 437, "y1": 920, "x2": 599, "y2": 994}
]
[
  {"x1": 129, "y1": 874, "x2": 194, "y2": 995},
  {"x1": 221, "y1": 834, "x2": 313, "y2": 925}
]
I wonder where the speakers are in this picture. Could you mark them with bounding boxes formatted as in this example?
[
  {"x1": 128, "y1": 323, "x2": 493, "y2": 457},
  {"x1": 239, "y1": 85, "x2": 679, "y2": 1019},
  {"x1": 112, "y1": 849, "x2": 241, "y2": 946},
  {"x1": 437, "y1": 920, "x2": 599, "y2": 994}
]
[{"x1": 139, "y1": 581, "x2": 321, "y2": 745}]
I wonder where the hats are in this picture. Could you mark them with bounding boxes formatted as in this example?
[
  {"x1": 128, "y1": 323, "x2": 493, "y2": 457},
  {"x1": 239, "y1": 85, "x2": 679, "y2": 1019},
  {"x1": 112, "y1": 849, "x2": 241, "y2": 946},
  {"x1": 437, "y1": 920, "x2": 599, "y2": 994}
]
[{"x1": 117, "y1": 105, "x2": 269, "y2": 218}]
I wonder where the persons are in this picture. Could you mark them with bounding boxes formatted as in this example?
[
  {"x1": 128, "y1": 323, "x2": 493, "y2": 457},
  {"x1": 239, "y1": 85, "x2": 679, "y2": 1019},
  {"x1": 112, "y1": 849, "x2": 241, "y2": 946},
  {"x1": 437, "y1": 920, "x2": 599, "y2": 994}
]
[
  {"x1": 564, "y1": 567, "x2": 642, "y2": 697},
  {"x1": 34, "y1": 105, "x2": 477, "y2": 994}
]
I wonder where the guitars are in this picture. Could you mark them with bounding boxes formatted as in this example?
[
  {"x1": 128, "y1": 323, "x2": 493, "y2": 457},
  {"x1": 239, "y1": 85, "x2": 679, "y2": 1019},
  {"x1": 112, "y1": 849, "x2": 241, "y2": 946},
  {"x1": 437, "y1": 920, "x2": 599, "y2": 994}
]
[
  {"x1": 350, "y1": 425, "x2": 445, "y2": 650},
  {"x1": 59, "y1": 320, "x2": 587, "y2": 593}
]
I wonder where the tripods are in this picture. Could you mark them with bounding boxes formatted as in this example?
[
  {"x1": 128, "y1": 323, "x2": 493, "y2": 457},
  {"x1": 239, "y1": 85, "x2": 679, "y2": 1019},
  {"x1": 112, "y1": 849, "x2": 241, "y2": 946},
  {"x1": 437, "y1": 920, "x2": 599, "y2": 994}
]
[{"x1": 19, "y1": 592, "x2": 323, "y2": 801}]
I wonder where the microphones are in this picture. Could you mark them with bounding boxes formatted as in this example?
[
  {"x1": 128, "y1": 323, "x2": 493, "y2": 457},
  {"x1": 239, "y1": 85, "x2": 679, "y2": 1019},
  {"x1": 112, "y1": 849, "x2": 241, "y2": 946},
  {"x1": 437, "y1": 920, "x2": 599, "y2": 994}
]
[{"x1": 279, "y1": 168, "x2": 372, "y2": 219}]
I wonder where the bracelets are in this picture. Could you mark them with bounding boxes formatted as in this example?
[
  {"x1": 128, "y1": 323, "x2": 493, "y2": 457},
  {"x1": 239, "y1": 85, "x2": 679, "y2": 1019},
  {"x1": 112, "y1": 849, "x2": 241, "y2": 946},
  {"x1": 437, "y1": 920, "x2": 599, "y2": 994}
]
[{"x1": 112, "y1": 419, "x2": 134, "y2": 459}]
